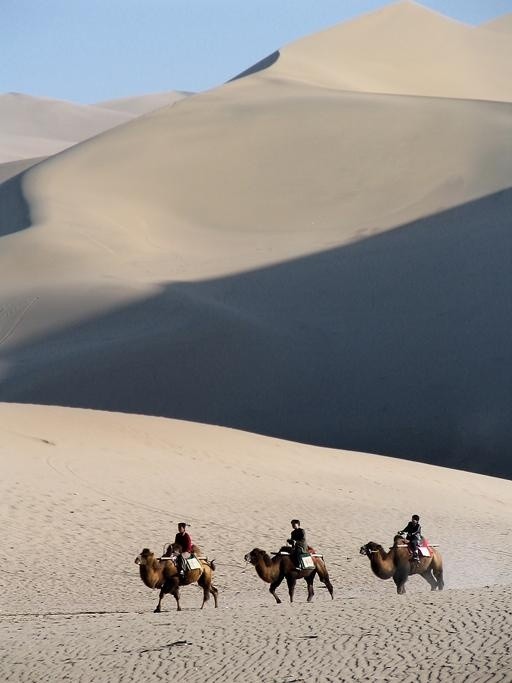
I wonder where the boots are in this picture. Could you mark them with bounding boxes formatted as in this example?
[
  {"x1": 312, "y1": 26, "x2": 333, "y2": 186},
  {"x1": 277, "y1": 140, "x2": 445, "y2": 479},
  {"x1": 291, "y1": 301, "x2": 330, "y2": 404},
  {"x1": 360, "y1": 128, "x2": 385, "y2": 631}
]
[
  {"x1": 296, "y1": 562, "x2": 302, "y2": 571},
  {"x1": 413, "y1": 552, "x2": 420, "y2": 560}
]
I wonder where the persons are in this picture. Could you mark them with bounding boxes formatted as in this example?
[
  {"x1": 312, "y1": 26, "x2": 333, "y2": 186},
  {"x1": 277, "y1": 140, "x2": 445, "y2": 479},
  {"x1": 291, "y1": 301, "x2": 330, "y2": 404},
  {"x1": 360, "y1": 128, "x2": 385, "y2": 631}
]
[
  {"x1": 291, "y1": 520, "x2": 306, "y2": 571},
  {"x1": 399, "y1": 515, "x2": 421, "y2": 560},
  {"x1": 175, "y1": 523, "x2": 191, "y2": 576}
]
